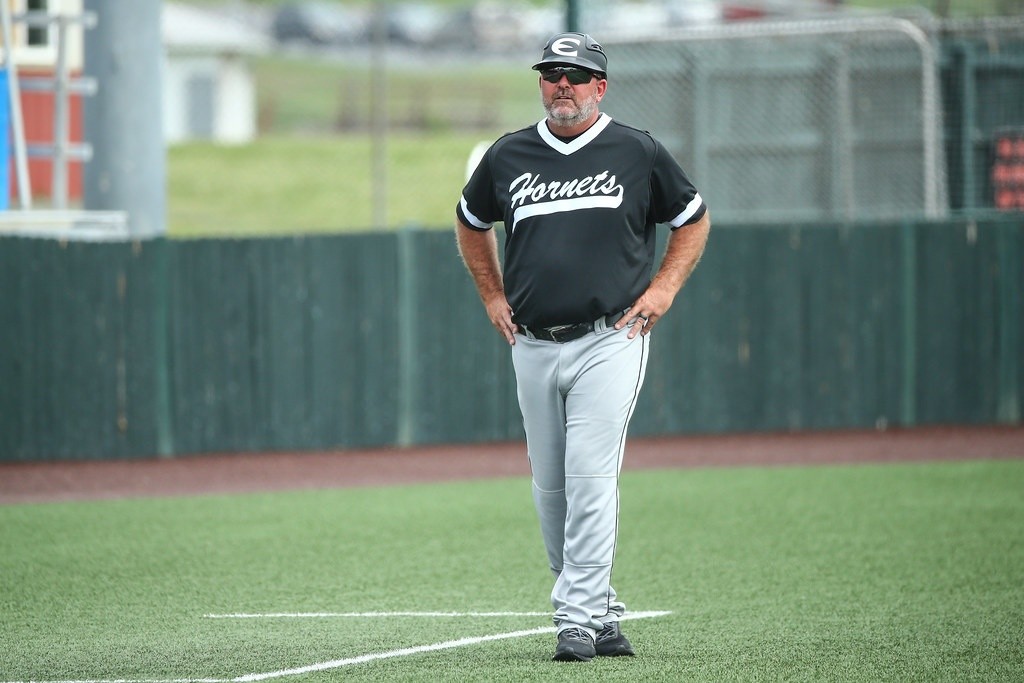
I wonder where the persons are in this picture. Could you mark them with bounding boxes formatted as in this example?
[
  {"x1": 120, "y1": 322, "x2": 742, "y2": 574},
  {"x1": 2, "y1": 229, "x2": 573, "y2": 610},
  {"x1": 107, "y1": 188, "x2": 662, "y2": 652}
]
[{"x1": 455, "y1": 32, "x2": 711, "y2": 661}]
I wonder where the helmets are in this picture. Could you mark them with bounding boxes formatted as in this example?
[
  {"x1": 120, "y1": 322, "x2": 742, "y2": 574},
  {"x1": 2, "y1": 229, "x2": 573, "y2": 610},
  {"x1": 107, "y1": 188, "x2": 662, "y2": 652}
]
[{"x1": 531, "y1": 32, "x2": 606, "y2": 77}]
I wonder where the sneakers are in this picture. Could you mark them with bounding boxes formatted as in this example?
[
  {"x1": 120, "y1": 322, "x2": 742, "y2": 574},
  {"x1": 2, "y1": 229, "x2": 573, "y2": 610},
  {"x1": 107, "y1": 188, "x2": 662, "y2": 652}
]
[
  {"x1": 594, "y1": 620, "x2": 635, "y2": 657},
  {"x1": 553, "y1": 626, "x2": 596, "y2": 662}
]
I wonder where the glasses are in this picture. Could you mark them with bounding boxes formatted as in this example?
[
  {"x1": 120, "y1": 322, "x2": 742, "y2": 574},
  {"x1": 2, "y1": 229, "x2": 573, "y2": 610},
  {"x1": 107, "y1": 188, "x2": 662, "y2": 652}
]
[{"x1": 539, "y1": 64, "x2": 602, "y2": 84}]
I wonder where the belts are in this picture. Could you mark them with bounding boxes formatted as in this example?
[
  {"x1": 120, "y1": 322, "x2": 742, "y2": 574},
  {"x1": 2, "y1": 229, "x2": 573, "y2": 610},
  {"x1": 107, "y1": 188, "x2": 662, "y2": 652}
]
[{"x1": 516, "y1": 310, "x2": 626, "y2": 343}]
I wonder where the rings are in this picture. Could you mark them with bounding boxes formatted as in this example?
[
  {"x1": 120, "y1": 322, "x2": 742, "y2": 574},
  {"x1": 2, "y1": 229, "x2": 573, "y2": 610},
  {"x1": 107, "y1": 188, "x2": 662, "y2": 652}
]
[{"x1": 638, "y1": 314, "x2": 647, "y2": 320}]
